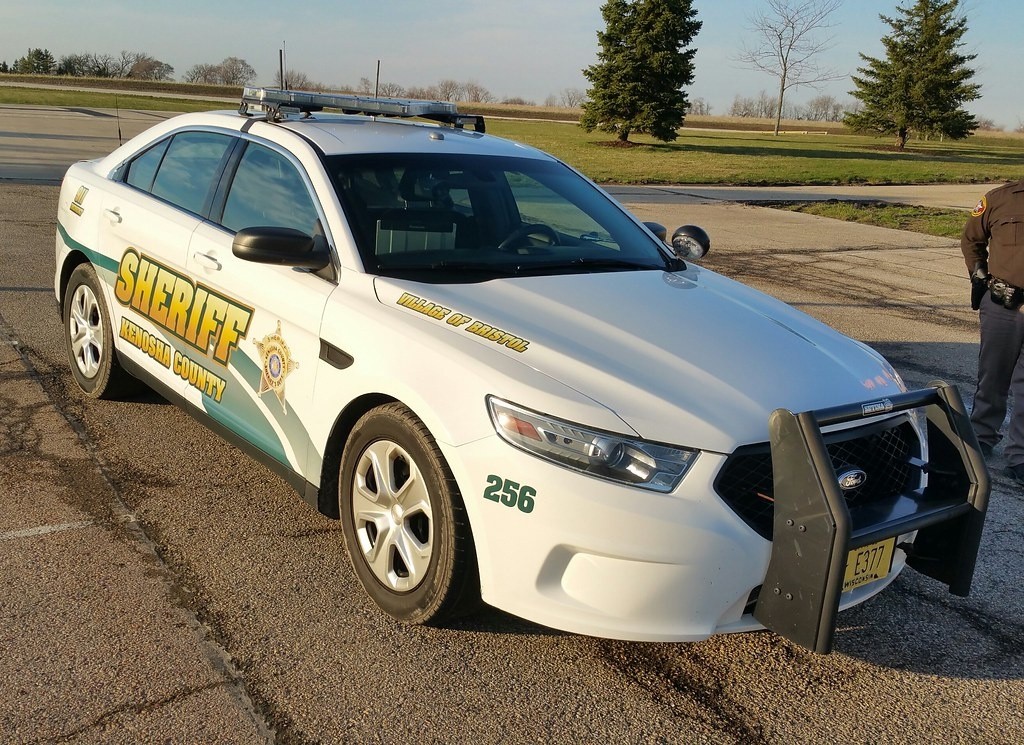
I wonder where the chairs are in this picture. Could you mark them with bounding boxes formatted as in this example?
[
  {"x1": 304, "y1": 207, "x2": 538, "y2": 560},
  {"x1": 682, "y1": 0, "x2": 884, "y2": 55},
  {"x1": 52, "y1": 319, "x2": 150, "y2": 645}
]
[{"x1": 384, "y1": 167, "x2": 479, "y2": 250}]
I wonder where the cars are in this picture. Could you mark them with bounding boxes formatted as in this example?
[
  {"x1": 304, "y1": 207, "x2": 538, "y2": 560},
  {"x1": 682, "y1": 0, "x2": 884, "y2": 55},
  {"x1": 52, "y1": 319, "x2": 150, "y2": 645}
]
[{"x1": 52, "y1": 50, "x2": 991, "y2": 655}]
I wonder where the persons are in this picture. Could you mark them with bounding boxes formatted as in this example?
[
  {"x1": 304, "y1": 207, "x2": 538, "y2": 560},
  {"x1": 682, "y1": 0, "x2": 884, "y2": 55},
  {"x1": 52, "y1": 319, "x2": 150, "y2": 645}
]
[{"x1": 961, "y1": 176, "x2": 1024, "y2": 484}]
